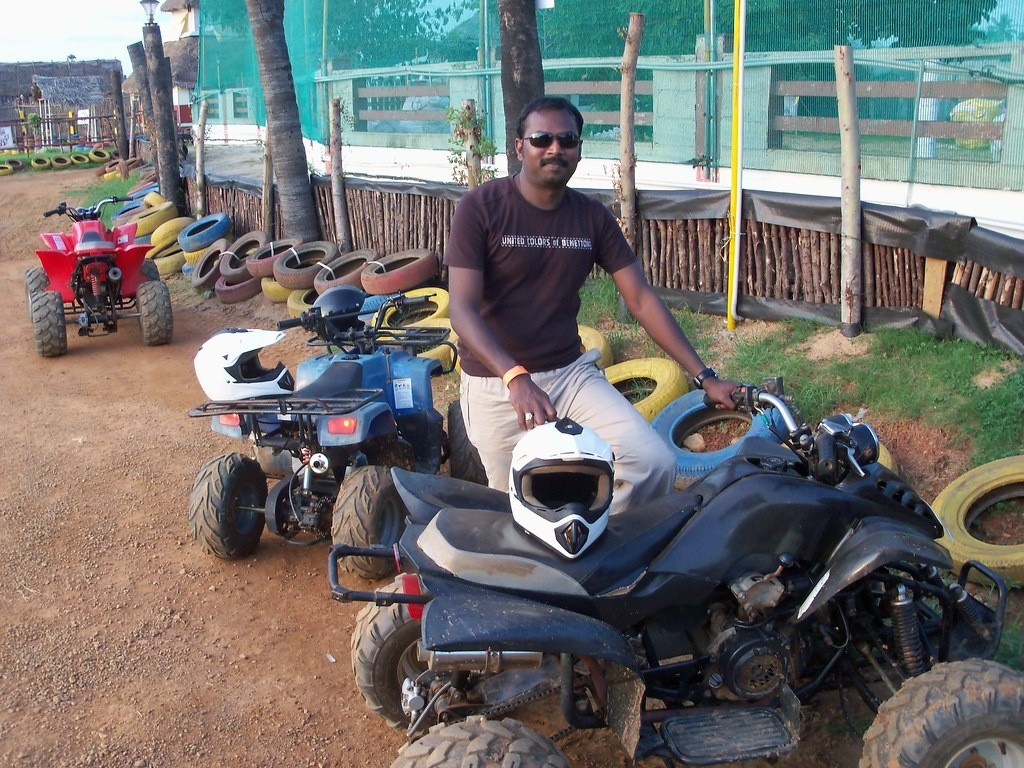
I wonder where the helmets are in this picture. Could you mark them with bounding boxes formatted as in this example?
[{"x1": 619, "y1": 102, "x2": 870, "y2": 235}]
[
  {"x1": 314, "y1": 284, "x2": 365, "y2": 337},
  {"x1": 507, "y1": 416, "x2": 615, "y2": 562}
]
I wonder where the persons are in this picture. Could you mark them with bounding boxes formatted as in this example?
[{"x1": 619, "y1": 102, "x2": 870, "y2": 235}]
[{"x1": 442, "y1": 97, "x2": 745, "y2": 517}]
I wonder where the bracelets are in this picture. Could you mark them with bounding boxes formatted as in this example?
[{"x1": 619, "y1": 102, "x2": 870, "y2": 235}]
[
  {"x1": 693, "y1": 367, "x2": 718, "y2": 389},
  {"x1": 502, "y1": 364, "x2": 529, "y2": 387}
]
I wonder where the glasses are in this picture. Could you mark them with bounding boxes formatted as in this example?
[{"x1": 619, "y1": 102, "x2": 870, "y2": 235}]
[{"x1": 524, "y1": 131, "x2": 580, "y2": 149}]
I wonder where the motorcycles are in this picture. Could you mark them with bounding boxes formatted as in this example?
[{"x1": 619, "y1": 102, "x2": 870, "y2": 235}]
[
  {"x1": 23, "y1": 195, "x2": 172, "y2": 358},
  {"x1": 329, "y1": 375, "x2": 1023, "y2": 768},
  {"x1": 188, "y1": 282, "x2": 492, "y2": 579}
]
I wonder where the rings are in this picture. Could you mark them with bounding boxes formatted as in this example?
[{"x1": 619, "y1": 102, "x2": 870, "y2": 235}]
[{"x1": 525, "y1": 413, "x2": 533, "y2": 420}]
